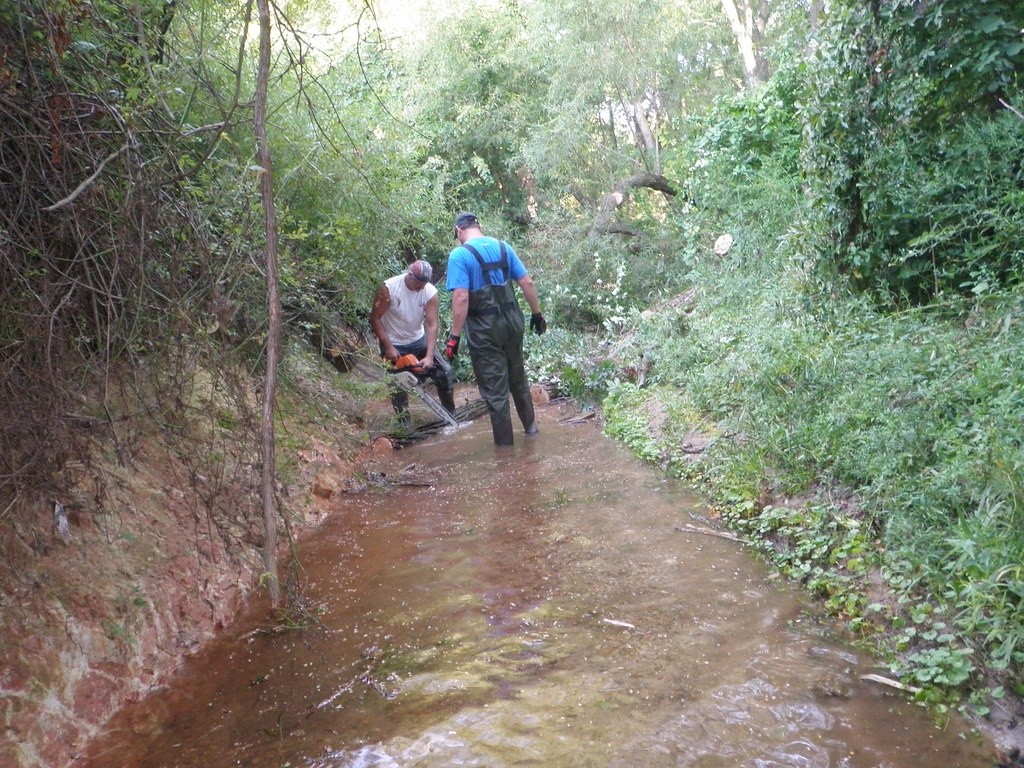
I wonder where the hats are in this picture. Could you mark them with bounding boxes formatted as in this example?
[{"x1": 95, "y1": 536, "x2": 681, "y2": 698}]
[
  {"x1": 453, "y1": 212, "x2": 479, "y2": 241},
  {"x1": 411, "y1": 260, "x2": 432, "y2": 281}
]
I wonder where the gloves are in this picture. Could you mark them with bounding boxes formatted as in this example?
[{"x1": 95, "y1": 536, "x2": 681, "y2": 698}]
[
  {"x1": 445, "y1": 334, "x2": 460, "y2": 363},
  {"x1": 530, "y1": 312, "x2": 547, "y2": 335}
]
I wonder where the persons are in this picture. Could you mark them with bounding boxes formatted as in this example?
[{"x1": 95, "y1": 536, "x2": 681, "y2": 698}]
[
  {"x1": 445, "y1": 212, "x2": 548, "y2": 445},
  {"x1": 371, "y1": 260, "x2": 456, "y2": 424}
]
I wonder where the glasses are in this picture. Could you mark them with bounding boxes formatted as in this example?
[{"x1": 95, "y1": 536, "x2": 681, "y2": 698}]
[{"x1": 413, "y1": 273, "x2": 429, "y2": 283}]
